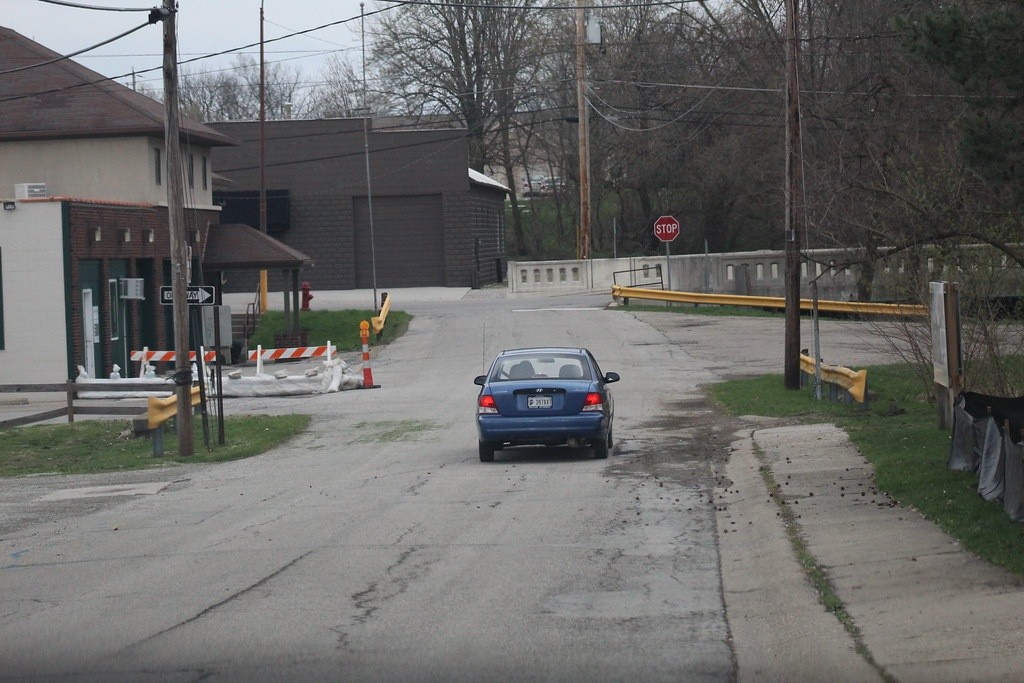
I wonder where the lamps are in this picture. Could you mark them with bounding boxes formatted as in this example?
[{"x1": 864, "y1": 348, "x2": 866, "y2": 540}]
[
  {"x1": 124, "y1": 228, "x2": 130, "y2": 242},
  {"x1": 88, "y1": 226, "x2": 101, "y2": 241},
  {"x1": 3, "y1": 202, "x2": 16, "y2": 210},
  {"x1": 191, "y1": 229, "x2": 201, "y2": 242},
  {"x1": 145, "y1": 229, "x2": 154, "y2": 242}
]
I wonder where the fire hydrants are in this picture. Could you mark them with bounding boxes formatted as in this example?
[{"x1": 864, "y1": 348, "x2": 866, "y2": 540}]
[{"x1": 300, "y1": 281, "x2": 314, "y2": 312}]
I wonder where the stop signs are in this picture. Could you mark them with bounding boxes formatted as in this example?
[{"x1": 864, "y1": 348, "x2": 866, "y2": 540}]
[{"x1": 654, "y1": 216, "x2": 679, "y2": 242}]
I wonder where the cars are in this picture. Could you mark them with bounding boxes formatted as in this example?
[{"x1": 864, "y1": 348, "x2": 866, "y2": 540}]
[{"x1": 473, "y1": 347, "x2": 620, "y2": 464}]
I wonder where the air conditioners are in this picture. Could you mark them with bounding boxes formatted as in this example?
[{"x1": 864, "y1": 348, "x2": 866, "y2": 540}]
[
  {"x1": 119, "y1": 277, "x2": 144, "y2": 297},
  {"x1": 14, "y1": 183, "x2": 46, "y2": 198}
]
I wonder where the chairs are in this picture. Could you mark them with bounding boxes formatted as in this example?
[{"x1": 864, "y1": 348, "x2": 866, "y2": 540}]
[
  {"x1": 509, "y1": 364, "x2": 531, "y2": 379},
  {"x1": 559, "y1": 364, "x2": 581, "y2": 377}
]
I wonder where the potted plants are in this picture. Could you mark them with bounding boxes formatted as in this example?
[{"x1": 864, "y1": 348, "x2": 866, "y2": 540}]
[{"x1": 274, "y1": 326, "x2": 308, "y2": 360}]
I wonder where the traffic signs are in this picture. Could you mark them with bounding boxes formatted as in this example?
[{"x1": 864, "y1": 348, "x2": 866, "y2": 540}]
[{"x1": 159, "y1": 285, "x2": 217, "y2": 308}]
